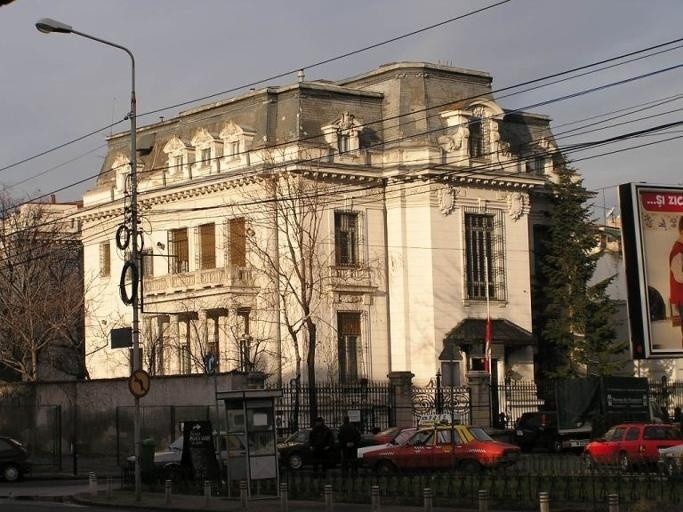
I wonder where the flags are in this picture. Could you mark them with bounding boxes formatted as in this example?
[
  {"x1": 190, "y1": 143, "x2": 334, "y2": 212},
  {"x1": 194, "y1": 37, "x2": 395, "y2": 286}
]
[{"x1": 482, "y1": 320, "x2": 492, "y2": 372}]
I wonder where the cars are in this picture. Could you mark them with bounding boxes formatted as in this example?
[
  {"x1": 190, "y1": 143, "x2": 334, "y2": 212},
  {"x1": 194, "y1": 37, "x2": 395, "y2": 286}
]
[
  {"x1": 580, "y1": 422, "x2": 682, "y2": 469},
  {"x1": 363, "y1": 414, "x2": 521, "y2": 471},
  {"x1": 278, "y1": 428, "x2": 326, "y2": 474},
  {"x1": 364, "y1": 426, "x2": 407, "y2": 445},
  {"x1": 123, "y1": 428, "x2": 252, "y2": 484}
]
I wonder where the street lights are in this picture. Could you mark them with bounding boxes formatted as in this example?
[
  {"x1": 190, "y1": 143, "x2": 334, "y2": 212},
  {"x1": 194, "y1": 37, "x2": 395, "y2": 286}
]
[{"x1": 33, "y1": 16, "x2": 145, "y2": 502}]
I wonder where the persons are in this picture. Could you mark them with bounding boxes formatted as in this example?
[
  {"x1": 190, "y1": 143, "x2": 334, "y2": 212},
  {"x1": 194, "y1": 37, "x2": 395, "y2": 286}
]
[
  {"x1": 307, "y1": 417, "x2": 334, "y2": 477},
  {"x1": 338, "y1": 415, "x2": 361, "y2": 477},
  {"x1": 670, "y1": 214, "x2": 683, "y2": 335}
]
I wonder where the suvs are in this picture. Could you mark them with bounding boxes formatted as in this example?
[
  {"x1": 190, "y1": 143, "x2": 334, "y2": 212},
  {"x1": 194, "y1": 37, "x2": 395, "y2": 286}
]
[
  {"x1": 512, "y1": 410, "x2": 582, "y2": 452},
  {"x1": 0, "y1": 436, "x2": 32, "y2": 482}
]
